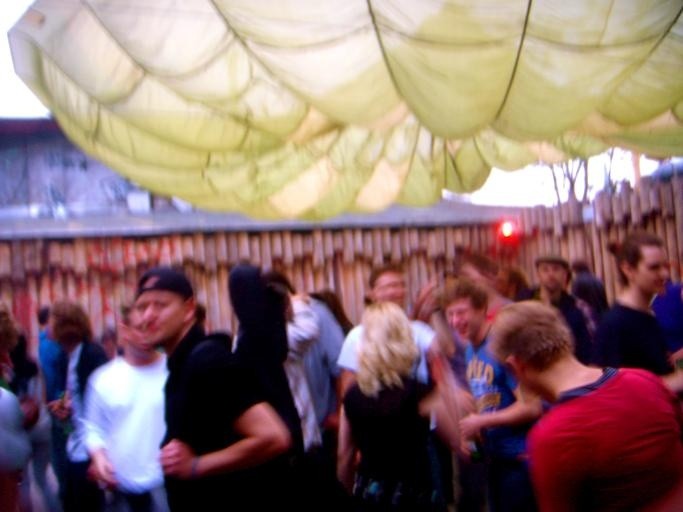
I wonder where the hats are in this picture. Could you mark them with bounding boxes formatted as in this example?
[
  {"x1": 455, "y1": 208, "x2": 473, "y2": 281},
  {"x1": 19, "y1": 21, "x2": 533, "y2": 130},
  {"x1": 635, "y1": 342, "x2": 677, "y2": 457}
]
[
  {"x1": 135, "y1": 267, "x2": 193, "y2": 301},
  {"x1": 535, "y1": 252, "x2": 570, "y2": 272}
]
